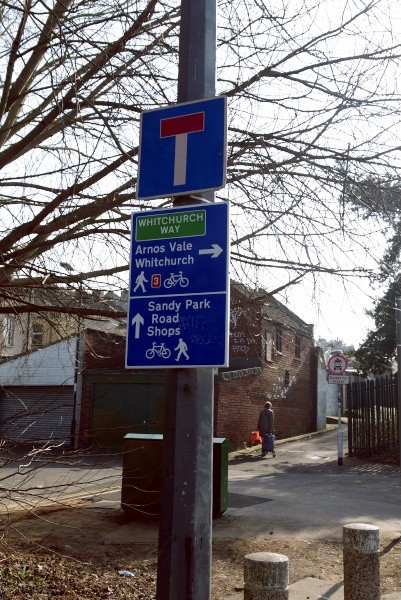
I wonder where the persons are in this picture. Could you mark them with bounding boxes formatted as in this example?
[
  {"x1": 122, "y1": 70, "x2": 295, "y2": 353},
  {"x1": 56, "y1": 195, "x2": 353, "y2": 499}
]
[{"x1": 256, "y1": 401, "x2": 276, "y2": 458}]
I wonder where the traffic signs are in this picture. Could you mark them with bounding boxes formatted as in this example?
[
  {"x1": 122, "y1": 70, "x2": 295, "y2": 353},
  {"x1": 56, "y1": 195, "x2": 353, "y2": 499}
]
[{"x1": 125, "y1": 204, "x2": 230, "y2": 369}]
